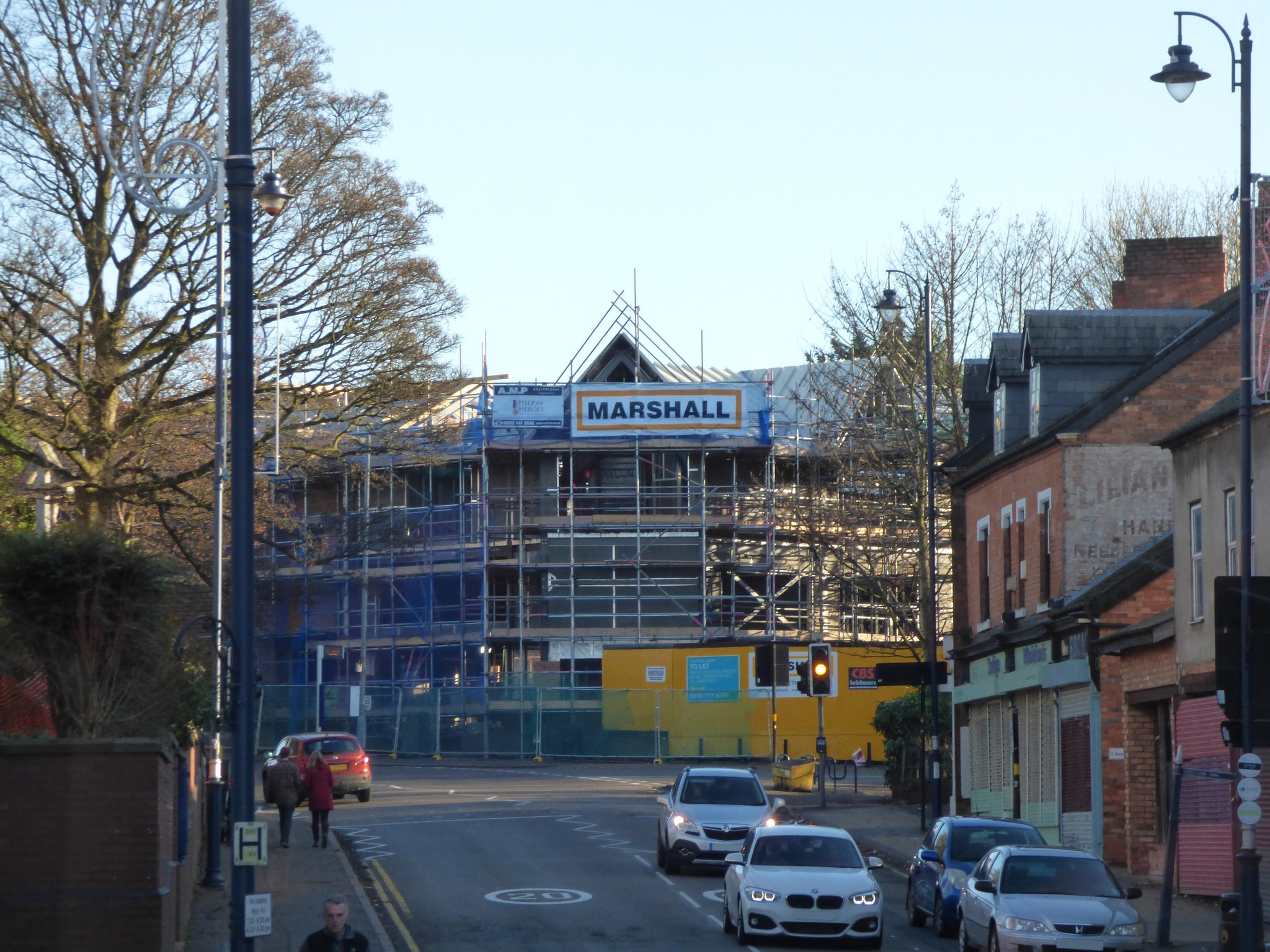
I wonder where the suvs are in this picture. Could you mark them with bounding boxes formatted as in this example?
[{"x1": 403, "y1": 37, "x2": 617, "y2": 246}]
[
  {"x1": 905, "y1": 812, "x2": 1048, "y2": 945},
  {"x1": 262, "y1": 729, "x2": 373, "y2": 808},
  {"x1": 656, "y1": 766, "x2": 786, "y2": 877}
]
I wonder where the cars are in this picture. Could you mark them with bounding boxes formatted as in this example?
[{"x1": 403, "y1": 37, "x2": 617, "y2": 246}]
[
  {"x1": 957, "y1": 842, "x2": 1148, "y2": 952},
  {"x1": 719, "y1": 819, "x2": 888, "y2": 949}
]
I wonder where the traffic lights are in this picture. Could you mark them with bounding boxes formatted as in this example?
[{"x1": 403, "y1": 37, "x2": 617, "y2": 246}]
[
  {"x1": 808, "y1": 642, "x2": 831, "y2": 696},
  {"x1": 797, "y1": 657, "x2": 811, "y2": 695}
]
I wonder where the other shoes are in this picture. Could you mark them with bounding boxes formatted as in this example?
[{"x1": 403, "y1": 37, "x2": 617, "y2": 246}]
[
  {"x1": 321, "y1": 842, "x2": 327, "y2": 848},
  {"x1": 312, "y1": 842, "x2": 319, "y2": 848},
  {"x1": 280, "y1": 842, "x2": 290, "y2": 849}
]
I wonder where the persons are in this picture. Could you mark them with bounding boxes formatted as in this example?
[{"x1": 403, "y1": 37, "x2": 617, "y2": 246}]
[
  {"x1": 269, "y1": 746, "x2": 302, "y2": 847},
  {"x1": 296, "y1": 896, "x2": 373, "y2": 952},
  {"x1": 305, "y1": 750, "x2": 334, "y2": 849}
]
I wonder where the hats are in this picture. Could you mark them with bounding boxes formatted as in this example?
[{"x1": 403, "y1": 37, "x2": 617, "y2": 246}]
[{"x1": 279, "y1": 746, "x2": 291, "y2": 754}]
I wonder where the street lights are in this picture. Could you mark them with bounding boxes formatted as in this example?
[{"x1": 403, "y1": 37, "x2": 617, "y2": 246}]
[
  {"x1": 1153, "y1": 0, "x2": 1270, "y2": 951},
  {"x1": 221, "y1": 12, "x2": 296, "y2": 950},
  {"x1": 871, "y1": 263, "x2": 945, "y2": 826}
]
